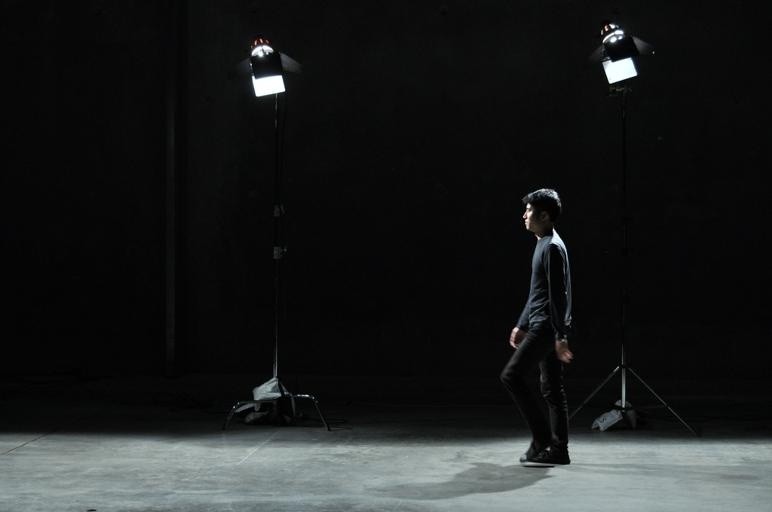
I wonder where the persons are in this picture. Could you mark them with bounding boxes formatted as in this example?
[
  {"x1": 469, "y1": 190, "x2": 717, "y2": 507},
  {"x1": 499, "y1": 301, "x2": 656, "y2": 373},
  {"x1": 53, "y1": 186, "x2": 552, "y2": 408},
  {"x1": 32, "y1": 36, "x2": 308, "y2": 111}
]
[{"x1": 500, "y1": 189, "x2": 574, "y2": 466}]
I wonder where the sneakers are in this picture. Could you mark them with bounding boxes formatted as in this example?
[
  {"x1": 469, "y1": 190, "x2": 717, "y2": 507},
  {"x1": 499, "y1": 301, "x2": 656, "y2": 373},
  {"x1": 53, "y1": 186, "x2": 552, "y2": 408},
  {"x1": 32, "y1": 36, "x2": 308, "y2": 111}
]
[{"x1": 519, "y1": 439, "x2": 570, "y2": 467}]
